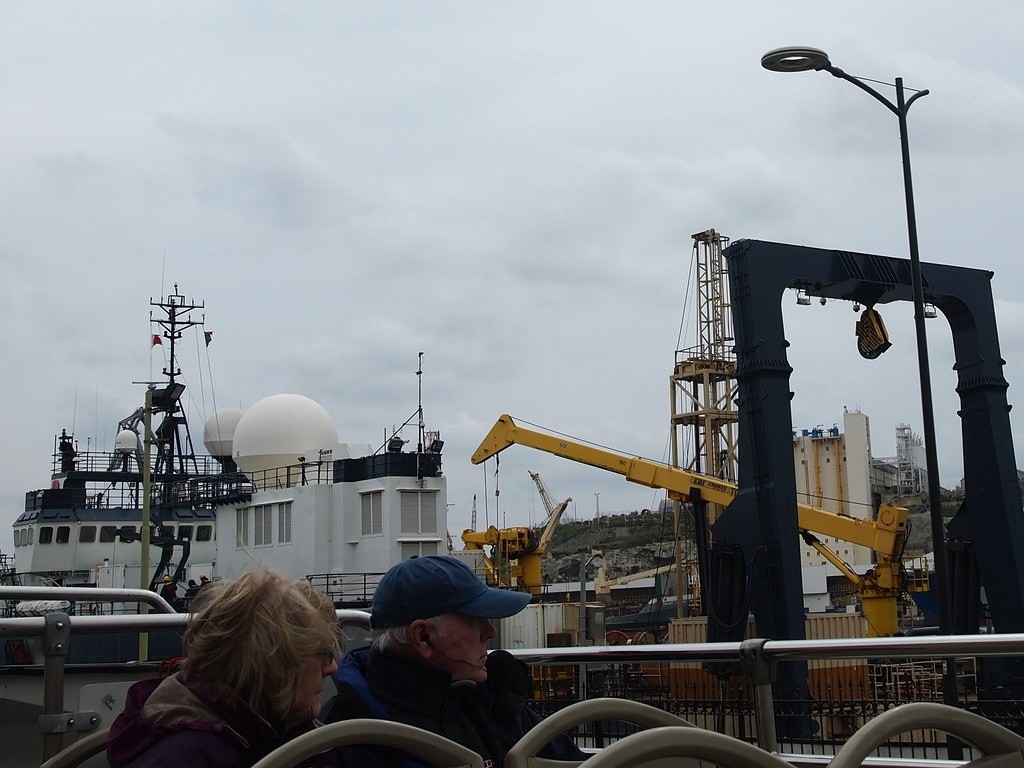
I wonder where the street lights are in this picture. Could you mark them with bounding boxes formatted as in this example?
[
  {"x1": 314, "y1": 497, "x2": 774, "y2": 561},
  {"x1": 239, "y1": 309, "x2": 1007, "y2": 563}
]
[{"x1": 760, "y1": 46, "x2": 962, "y2": 760}]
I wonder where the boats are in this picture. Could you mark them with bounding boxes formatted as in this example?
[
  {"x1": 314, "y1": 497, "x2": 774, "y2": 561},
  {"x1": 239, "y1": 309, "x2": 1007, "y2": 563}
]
[{"x1": 0, "y1": 282, "x2": 554, "y2": 668}]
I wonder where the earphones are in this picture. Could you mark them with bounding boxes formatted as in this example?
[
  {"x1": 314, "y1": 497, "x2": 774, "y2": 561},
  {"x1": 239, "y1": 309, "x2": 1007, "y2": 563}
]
[{"x1": 421, "y1": 634, "x2": 434, "y2": 649}]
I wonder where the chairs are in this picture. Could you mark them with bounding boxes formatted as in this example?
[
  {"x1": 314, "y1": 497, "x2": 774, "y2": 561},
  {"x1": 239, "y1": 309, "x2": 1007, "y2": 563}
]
[{"x1": 39, "y1": 697, "x2": 1024, "y2": 767}]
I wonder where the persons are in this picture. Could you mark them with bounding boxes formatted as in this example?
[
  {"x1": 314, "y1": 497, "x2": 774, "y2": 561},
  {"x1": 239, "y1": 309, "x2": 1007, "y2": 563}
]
[
  {"x1": 185, "y1": 579, "x2": 201, "y2": 597},
  {"x1": 198, "y1": 574, "x2": 212, "y2": 586},
  {"x1": 161, "y1": 576, "x2": 177, "y2": 604},
  {"x1": 319, "y1": 554, "x2": 597, "y2": 768},
  {"x1": 107, "y1": 570, "x2": 339, "y2": 768}
]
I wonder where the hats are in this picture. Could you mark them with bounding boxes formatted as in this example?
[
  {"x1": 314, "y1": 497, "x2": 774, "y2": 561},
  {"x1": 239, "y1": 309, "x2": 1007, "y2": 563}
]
[{"x1": 369, "y1": 555, "x2": 532, "y2": 629}]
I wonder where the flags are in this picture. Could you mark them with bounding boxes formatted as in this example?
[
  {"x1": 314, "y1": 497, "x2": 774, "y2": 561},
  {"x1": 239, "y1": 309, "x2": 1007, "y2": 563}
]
[
  {"x1": 205, "y1": 333, "x2": 211, "y2": 347},
  {"x1": 153, "y1": 337, "x2": 162, "y2": 345}
]
